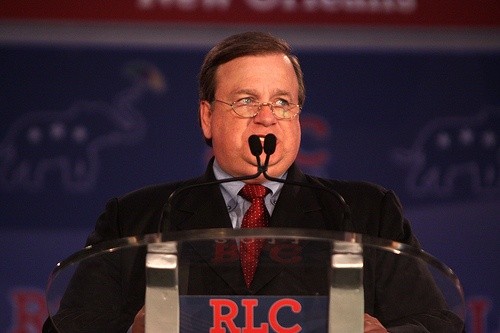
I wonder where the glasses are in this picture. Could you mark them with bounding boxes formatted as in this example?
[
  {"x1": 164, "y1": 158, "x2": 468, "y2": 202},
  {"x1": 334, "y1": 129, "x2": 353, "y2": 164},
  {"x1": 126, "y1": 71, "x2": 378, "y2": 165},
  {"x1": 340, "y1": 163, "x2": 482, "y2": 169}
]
[{"x1": 212, "y1": 99, "x2": 302, "y2": 120}]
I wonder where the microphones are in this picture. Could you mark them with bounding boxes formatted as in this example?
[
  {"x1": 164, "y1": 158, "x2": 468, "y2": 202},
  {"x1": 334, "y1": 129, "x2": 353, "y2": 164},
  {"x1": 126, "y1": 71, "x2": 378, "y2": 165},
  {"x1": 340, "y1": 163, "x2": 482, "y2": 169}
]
[
  {"x1": 264, "y1": 134, "x2": 349, "y2": 232},
  {"x1": 157, "y1": 135, "x2": 262, "y2": 233}
]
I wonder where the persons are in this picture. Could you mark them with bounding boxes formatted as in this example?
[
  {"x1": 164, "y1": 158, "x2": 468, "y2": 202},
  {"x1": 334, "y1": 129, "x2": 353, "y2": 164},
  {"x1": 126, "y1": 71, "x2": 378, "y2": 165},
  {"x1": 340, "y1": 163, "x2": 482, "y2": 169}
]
[{"x1": 43, "y1": 32, "x2": 467, "y2": 333}]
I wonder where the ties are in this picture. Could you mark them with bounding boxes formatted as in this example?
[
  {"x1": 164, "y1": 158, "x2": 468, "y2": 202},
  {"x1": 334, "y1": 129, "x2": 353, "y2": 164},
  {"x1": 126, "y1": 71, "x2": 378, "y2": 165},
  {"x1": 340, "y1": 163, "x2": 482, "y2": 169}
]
[{"x1": 237, "y1": 182, "x2": 274, "y2": 289}]
